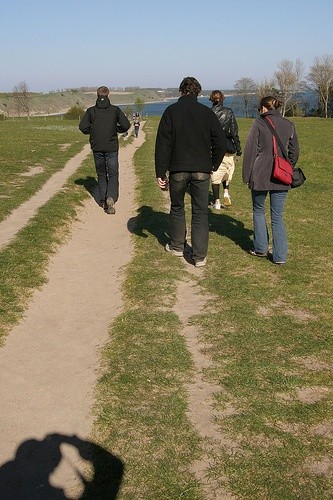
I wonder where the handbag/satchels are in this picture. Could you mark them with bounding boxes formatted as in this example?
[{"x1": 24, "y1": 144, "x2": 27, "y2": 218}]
[
  {"x1": 292, "y1": 167, "x2": 306, "y2": 188},
  {"x1": 273, "y1": 156, "x2": 294, "y2": 184}
]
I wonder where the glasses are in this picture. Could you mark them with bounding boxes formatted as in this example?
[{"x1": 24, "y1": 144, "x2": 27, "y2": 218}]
[{"x1": 258, "y1": 107, "x2": 263, "y2": 112}]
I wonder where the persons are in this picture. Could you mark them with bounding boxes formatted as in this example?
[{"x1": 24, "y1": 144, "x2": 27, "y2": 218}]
[
  {"x1": 133, "y1": 112, "x2": 141, "y2": 138},
  {"x1": 242, "y1": 96, "x2": 299, "y2": 264},
  {"x1": 78, "y1": 86, "x2": 131, "y2": 214},
  {"x1": 155, "y1": 77, "x2": 226, "y2": 266},
  {"x1": 208, "y1": 90, "x2": 242, "y2": 210}
]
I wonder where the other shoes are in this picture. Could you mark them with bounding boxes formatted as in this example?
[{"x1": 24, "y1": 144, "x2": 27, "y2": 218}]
[
  {"x1": 166, "y1": 242, "x2": 182, "y2": 256},
  {"x1": 107, "y1": 197, "x2": 116, "y2": 214},
  {"x1": 250, "y1": 248, "x2": 267, "y2": 256},
  {"x1": 195, "y1": 257, "x2": 206, "y2": 267},
  {"x1": 224, "y1": 193, "x2": 231, "y2": 206},
  {"x1": 213, "y1": 204, "x2": 221, "y2": 209},
  {"x1": 102, "y1": 201, "x2": 106, "y2": 209},
  {"x1": 275, "y1": 260, "x2": 287, "y2": 263}
]
